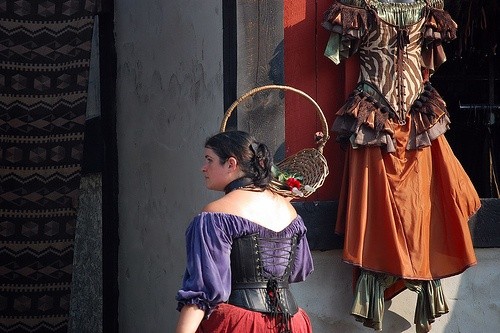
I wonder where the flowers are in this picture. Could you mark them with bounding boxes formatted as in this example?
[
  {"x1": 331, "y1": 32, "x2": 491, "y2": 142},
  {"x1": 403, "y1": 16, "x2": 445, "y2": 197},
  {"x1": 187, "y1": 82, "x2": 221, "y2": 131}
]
[{"x1": 270, "y1": 164, "x2": 303, "y2": 191}]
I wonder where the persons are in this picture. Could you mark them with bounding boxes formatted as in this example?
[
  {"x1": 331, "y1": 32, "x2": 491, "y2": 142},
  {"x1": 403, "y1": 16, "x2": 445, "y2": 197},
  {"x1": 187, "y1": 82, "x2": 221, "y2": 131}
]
[{"x1": 176, "y1": 130, "x2": 314, "y2": 333}]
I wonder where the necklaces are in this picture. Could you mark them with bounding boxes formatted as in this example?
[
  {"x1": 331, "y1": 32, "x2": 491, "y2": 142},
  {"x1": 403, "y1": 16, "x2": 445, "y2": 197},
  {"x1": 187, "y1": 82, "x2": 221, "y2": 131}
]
[{"x1": 236, "y1": 183, "x2": 258, "y2": 189}]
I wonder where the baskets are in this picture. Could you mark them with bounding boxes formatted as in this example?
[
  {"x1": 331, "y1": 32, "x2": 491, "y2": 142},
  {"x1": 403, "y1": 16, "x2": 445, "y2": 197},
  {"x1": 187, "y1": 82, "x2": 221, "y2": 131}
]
[{"x1": 220, "y1": 85, "x2": 330, "y2": 200}]
